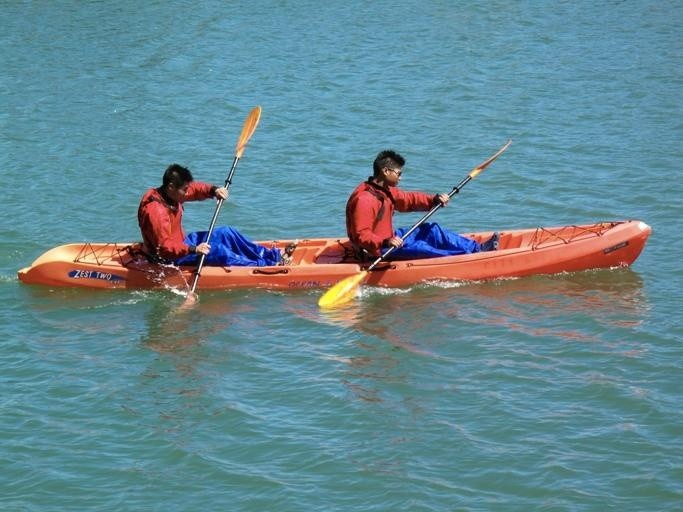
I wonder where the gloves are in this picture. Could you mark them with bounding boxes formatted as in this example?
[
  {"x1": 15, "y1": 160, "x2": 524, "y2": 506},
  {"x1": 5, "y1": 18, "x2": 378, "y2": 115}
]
[
  {"x1": 19, "y1": 220, "x2": 655, "y2": 294},
  {"x1": 22, "y1": 265, "x2": 646, "y2": 343}
]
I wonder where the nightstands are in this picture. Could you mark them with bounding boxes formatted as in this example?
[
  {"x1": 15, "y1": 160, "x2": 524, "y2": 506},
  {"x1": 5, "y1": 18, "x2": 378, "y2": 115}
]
[
  {"x1": 179, "y1": 105, "x2": 262, "y2": 309},
  {"x1": 317, "y1": 141, "x2": 513, "y2": 310}
]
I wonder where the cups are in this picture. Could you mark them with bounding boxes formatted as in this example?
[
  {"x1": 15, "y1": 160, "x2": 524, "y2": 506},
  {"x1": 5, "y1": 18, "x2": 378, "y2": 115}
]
[{"x1": 390, "y1": 169, "x2": 401, "y2": 176}]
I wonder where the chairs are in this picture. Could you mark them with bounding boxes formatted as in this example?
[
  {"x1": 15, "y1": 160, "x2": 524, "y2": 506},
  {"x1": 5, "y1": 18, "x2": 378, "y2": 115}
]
[{"x1": 483, "y1": 232, "x2": 499, "y2": 251}]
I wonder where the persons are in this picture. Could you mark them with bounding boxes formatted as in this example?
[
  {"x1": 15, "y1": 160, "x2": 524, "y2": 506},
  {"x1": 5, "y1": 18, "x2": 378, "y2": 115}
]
[
  {"x1": 138, "y1": 164, "x2": 293, "y2": 267},
  {"x1": 346, "y1": 151, "x2": 498, "y2": 264}
]
